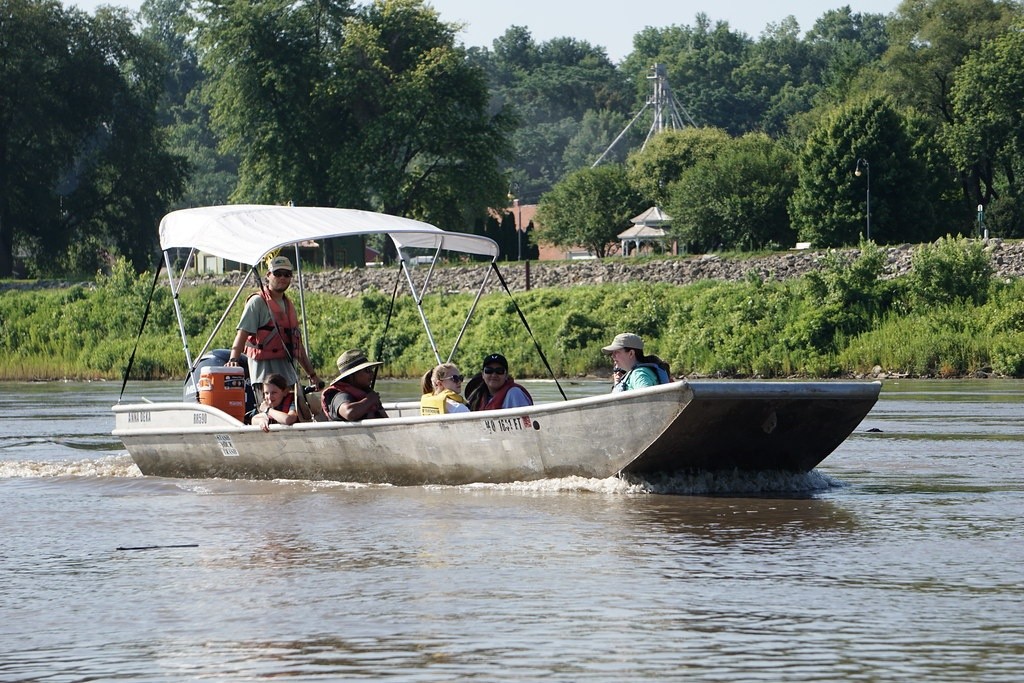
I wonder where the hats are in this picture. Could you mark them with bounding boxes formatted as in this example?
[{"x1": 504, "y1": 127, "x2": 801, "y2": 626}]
[
  {"x1": 330, "y1": 349, "x2": 384, "y2": 386},
  {"x1": 268, "y1": 256, "x2": 293, "y2": 272},
  {"x1": 601, "y1": 333, "x2": 643, "y2": 355},
  {"x1": 482, "y1": 353, "x2": 508, "y2": 372}
]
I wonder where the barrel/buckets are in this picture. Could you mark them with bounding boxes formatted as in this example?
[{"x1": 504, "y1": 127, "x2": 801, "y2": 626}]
[{"x1": 196, "y1": 366, "x2": 245, "y2": 423}]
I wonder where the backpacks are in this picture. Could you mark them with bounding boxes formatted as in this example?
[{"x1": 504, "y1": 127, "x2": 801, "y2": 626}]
[{"x1": 612, "y1": 355, "x2": 671, "y2": 391}]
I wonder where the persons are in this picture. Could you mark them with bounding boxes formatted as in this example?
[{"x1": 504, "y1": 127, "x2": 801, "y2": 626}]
[
  {"x1": 601, "y1": 333, "x2": 671, "y2": 394},
  {"x1": 321, "y1": 349, "x2": 390, "y2": 422},
  {"x1": 251, "y1": 374, "x2": 300, "y2": 432},
  {"x1": 464, "y1": 354, "x2": 534, "y2": 411},
  {"x1": 613, "y1": 362, "x2": 627, "y2": 384},
  {"x1": 420, "y1": 363, "x2": 470, "y2": 415},
  {"x1": 222, "y1": 256, "x2": 326, "y2": 421}
]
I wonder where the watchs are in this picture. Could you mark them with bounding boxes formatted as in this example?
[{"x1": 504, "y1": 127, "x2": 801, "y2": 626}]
[
  {"x1": 264, "y1": 407, "x2": 271, "y2": 414},
  {"x1": 229, "y1": 358, "x2": 239, "y2": 362}
]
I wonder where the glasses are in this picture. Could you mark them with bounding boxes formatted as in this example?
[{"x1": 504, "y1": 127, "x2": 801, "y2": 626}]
[
  {"x1": 364, "y1": 366, "x2": 376, "y2": 375},
  {"x1": 272, "y1": 269, "x2": 293, "y2": 278},
  {"x1": 613, "y1": 367, "x2": 626, "y2": 374},
  {"x1": 484, "y1": 366, "x2": 505, "y2": 375},
  {"x1": 440, "y1": 375, "x2": 463, "y2": 383}
]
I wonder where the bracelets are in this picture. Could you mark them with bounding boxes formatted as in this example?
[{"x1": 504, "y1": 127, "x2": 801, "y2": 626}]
[{"x1": 307, "y1": 373, "x2": 316, "y2": 379}]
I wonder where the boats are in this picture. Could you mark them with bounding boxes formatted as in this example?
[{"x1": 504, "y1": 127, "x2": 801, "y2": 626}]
[{"x1": 111, "y1": 200, "x2": 882, "y2": 486}]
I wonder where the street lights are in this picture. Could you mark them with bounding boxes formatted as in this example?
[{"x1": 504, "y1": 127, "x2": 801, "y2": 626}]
[{"x1": 855, "y1": 158, "x2": 869, "y2": 240}]
